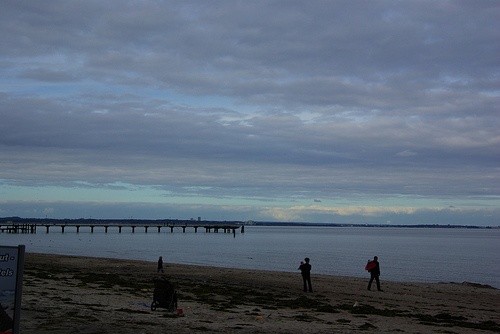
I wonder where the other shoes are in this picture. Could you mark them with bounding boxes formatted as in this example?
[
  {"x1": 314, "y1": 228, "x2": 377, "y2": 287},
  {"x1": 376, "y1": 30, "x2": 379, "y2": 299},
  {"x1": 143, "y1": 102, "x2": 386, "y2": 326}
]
[
  {"x1": 377, "y1": 288, "x2": 383, "y2": 292},
  {"x1": 303, "y1": 290, "x2": 312, "y2": 292},
  {"x1": 367, "y1": 289, "x2": 370, "y2": 291}
]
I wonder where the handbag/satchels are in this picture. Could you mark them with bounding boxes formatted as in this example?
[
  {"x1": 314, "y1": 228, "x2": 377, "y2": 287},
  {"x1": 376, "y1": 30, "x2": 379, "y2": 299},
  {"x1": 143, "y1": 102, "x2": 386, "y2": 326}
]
[{"x1": 365, "y1": 262, "x2": 376, "y2": 271}]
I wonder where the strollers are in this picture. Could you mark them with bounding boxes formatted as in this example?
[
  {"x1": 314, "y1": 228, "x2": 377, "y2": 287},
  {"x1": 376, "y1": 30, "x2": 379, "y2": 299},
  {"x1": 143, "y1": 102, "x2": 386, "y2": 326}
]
[{"x1": 148, "y1": 277, "x2": 179, "y2": 312}]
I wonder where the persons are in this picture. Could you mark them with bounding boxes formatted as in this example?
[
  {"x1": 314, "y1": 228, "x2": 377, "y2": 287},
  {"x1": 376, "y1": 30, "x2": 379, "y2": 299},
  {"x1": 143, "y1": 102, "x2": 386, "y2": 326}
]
[
  {"x1": 367, "y1": 256, "x2": 384, "y2": 291},
  {"x1": 157, "y1": 255, "x2": 164, "y2": 274},
  {"x1": 299, "y1": 257, "x2": 313, "y2": 292},
  {"x1": 297, "y1": 262, "x2": 304, "y2": 270}
]
